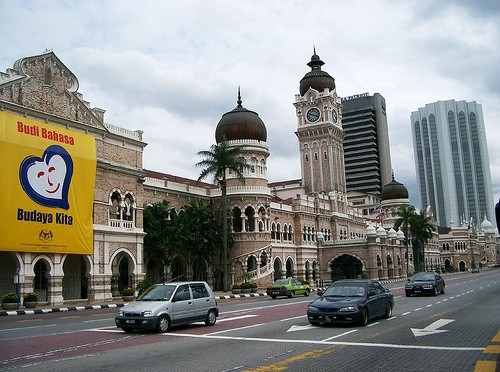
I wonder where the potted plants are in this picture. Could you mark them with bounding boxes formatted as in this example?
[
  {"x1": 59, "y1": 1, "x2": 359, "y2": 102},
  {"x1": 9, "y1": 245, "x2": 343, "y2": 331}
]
[
  {"x1": 121, "y1": 287, "x2": 136, "y2": 302},
  {"x1": 1, "y1": 292, "x2": 20, "y2": 310},
  {"x1": 23, "y1": 292, "x2": 38, "y2": 308},
  {"x1": 231, "y1": 282, "x2": 258, "y2": 294}
]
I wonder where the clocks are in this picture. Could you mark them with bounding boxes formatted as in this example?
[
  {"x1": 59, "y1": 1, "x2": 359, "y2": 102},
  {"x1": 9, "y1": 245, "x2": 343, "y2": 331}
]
[
  {"x1": 331, "y1": 108, "x2": 338, "y2": 124},
  {"x1": 305, "y1": 107, "x2": 321, "y2": 124}
]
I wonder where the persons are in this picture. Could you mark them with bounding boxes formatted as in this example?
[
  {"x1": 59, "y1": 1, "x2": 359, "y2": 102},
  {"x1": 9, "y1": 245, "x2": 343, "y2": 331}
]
[{"x1": 358, "y1": 287, "x2": 363, "y2": 296}]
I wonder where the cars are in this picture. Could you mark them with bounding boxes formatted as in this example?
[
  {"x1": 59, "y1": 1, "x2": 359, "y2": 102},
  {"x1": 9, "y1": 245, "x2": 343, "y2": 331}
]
[
  {"x1": 307, "y1": 279, "x2": 394, "y2": 327},
  {"x1": 472, "y1": 267, "x2": 480, "y2": 273},
  {"x1": 404, "y1": 272, "x2": 445, "y2": 298},
  {"x1": 266, "y1": 276, "x2": 311, "y2": 299}
]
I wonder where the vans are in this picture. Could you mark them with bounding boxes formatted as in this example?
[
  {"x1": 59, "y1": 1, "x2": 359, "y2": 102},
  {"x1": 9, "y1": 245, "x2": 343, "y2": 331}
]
[{"x1": 115, "y1": 281, "x2": 220, "y2": 333}]
